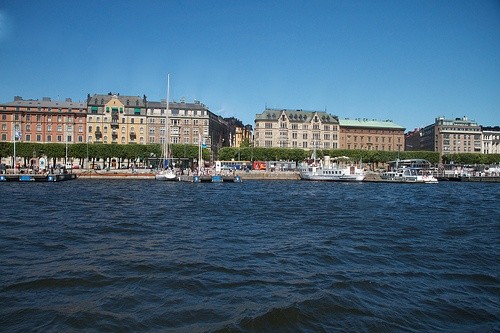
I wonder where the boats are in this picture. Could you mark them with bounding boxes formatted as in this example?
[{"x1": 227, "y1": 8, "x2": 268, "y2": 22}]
[
  {"x1": 155, "y1": 72, "x2": 178, "y2": 181},
  {"x1": 299, "y1": 165, "x2": 357, "y2": 181},
  {"x1": 380, "y1": 167, "x2": 438, "y2": 182}
]
